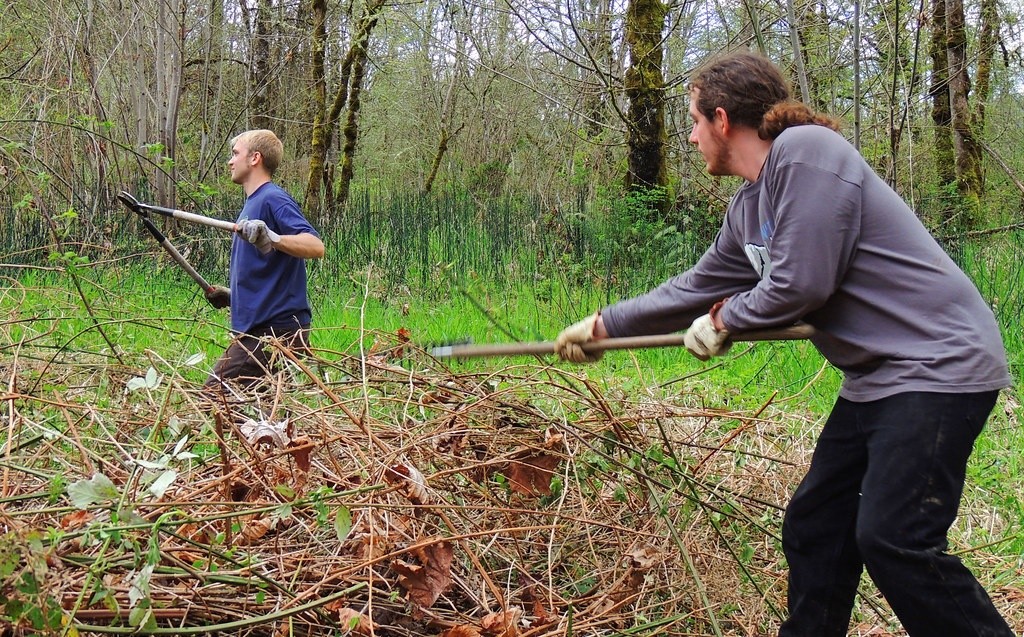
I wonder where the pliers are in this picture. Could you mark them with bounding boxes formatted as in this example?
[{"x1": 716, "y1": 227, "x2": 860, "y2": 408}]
[{"x1": 115, "y1": 190, "x2": 237, "y2": 293}]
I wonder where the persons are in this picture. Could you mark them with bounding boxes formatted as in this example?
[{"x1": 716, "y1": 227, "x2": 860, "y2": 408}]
[
  {"x1": 201, "y1": 129, "x2": 324, "y2": 432},
  {"x1": 554, "y1": 55, "x2": 1019, "y2": 637}
]
